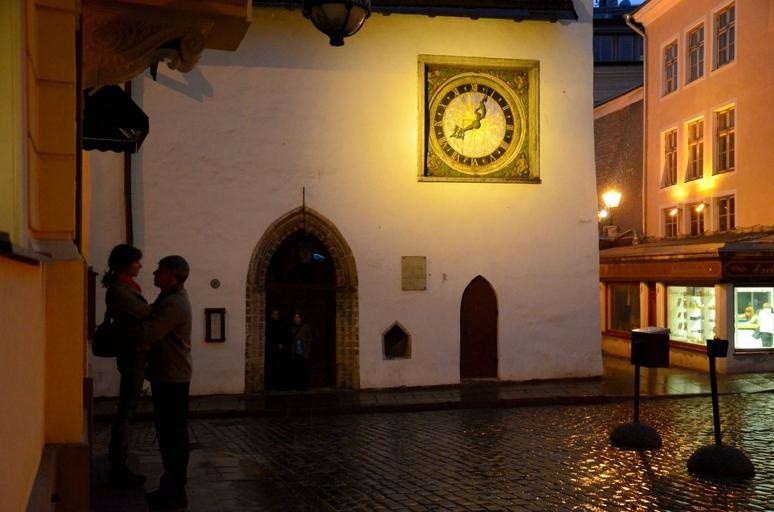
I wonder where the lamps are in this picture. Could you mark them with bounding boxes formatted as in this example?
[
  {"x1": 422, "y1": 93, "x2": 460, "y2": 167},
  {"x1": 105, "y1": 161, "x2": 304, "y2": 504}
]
[
  {"x1": 669, "y1": 207, "x2": 683, "y2": 216},
  {"x1": 602, "y1": 189, "x2": 622, "y2": 226},
  {"x1": 301, "y1": 0, "x2": 371, "y2": 47},
  {"x1": 695, "y1": 201, "x2": 709, "y2": 212}
]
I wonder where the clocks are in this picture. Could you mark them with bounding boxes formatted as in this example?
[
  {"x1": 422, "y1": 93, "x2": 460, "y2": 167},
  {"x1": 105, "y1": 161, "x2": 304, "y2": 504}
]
[{"x1": 416, "y1": 52, "x2": 542, "y2": 185}]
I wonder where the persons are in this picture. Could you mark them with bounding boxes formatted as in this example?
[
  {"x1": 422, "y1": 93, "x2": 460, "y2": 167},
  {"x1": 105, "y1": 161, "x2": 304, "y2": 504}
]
[
  {"x1": 290, "y1": 312, "x2": 314, "y2": 363},
  {"x1": 512, "y1": 152, "x2": 529, "y2": 177},
  {"x1": 101, "y1": 238, "x2": 155, "y2": 493},
  {"x1": 735, "y1": 306, "x2": 758, "y2": 325},
  {"x1": 756, "y1": 302, "x2": 774, "y2": 348},
  {"x1": 111, "y1": 254, "x2": 195, "y2": 511},
  {"x1": 267, "y1": 308, "x2": 286, "y2": 350}
]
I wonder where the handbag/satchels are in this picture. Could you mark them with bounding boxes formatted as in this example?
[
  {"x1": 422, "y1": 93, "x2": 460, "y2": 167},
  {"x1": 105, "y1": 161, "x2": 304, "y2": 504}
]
[{"x1": 92, "y1": 309, "x2": 124, "y2": 358}]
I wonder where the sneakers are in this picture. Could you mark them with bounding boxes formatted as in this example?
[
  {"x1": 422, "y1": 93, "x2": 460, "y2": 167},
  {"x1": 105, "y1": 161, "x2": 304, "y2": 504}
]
[
  {"x1": 108, "y1": 465, "x2": 148, "y2": 486},
  {"x1": 146, "y1": 477, "x2": 193, "y2": 511}
]
